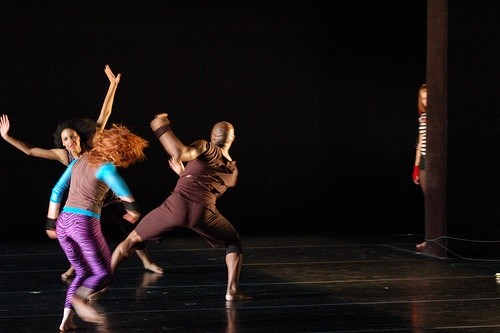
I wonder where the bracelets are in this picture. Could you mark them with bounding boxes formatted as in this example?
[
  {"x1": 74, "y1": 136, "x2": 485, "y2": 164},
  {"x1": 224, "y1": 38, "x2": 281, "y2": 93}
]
[{"x1": 154, "y1": 124, "x2": 171, "y2": 138}]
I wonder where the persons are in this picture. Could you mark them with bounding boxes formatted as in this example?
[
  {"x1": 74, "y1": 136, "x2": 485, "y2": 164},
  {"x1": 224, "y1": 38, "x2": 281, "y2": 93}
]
[
  {"x1": 413, "y1": 84, "x2": 428, "y2": 248},
  {"x1": 89, "y1": 114, "x2": 253, "y2": 300},
  {"x1": 0, "y1": 65, "x2": 165, "y2": 278},
  {"x1": 46, "y1": 124, "x2": 148, "y2": 331}
]
